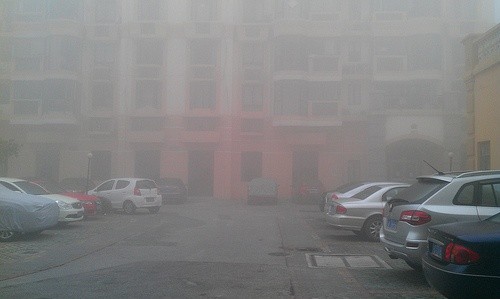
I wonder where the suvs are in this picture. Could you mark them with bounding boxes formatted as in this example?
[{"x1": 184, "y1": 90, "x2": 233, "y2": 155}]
[{"x1": 376, "y1": 167, "x2": 500, "y2": 274}]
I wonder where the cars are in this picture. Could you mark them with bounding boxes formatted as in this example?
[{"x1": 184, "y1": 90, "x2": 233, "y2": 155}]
[
  {"x1": 0, "y1": 178, "x2": 103, "y2": 241},
  {"x1": 87, "y1": 175, "x2": 186, "y2": 216},
  {"x1": 422, "y1": 212, "x2": 500, "y2": 299},
  {"x1": 321, "y1": 180, "x2": 412, "y2": 243}
]
